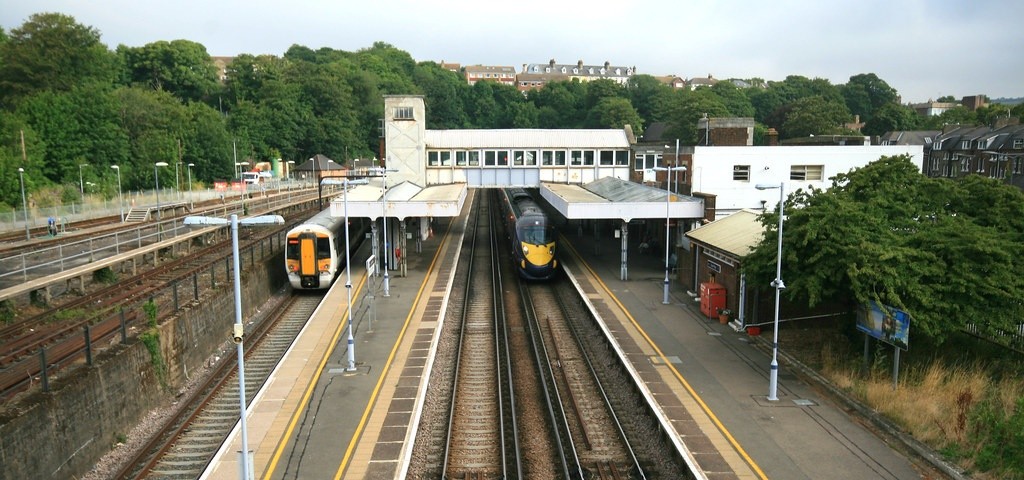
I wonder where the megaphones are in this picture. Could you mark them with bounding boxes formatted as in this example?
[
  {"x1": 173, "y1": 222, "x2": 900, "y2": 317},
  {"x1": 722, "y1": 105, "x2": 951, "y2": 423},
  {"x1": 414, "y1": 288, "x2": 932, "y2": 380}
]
[
  {"x1": 770, "y1": 278, "x2": 777, "y2": 288},
  {"x1": 233, "y1": 324, "x2": 243, "y2": 344},
  {"x1": 345, "y1": 281, "x2": 352, "y2": 288},
  {"x1": 778, "y1": 279, "x2": 786, "y2": 290}
]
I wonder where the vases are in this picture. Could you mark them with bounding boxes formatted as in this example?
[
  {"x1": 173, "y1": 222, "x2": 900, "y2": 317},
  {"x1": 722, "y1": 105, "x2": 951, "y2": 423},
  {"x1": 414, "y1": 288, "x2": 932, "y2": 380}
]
[{"x1": 718, "y1": 314, "x2": 729, "y2": 324}]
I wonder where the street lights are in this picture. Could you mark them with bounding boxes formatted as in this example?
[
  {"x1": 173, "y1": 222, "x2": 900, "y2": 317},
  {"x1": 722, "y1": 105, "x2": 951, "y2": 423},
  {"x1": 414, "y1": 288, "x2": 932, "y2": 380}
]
[
  {"x1": 319, "y1": 178, "x2": 371, "y2": 371},
  {"x1": 174, "y1": 162, "x2": 184, "y2": 203},
  {"x1": 308, "y1": 158, "x2": 316, "y2": 191},
  {"x1": 326, "y1": 160, "x2": 335, "y2": 180},
  {"x1": 286, "y1": 160, "x2": 294, "y2": 202},
  {"x1": 184, "y1": 213, "x2": 286, "y2": 480},
  {"x1": 652, "y1": 163, "x2": 688, "y2": 304},
  {"x1": 354, "y1": 158, "x2": 360, "y2": 182},
  {"x1": 366, "y1": 166, "x2": 399, "y2": 298},
  {"x1": 277, "y1": 158, "x2": 284, "y2": 194},
  {"x1": 236, "y1": 164, "x2": 242, "y2": 181},
  {"x1": 154, "y1": 162, "x2": 170, "y2": 243},
  {"x1": 109, "y1": 164, "x2": 125, "y2": 224},
  {"x1": 78, "y1": 163, "x2": 88, "y2": 213},
  {"x1": 187, "y1": 163, "x2": 197, "y2": 215},
  {"x1": 238, "y1": 161, "x2": 251, "y2": 213},
  {"x1": 754, "y1": 182, "x2": 787, "y2": 404},
  {"x1": 18, "y1": 167, "x2": 31, "y2": 242}
]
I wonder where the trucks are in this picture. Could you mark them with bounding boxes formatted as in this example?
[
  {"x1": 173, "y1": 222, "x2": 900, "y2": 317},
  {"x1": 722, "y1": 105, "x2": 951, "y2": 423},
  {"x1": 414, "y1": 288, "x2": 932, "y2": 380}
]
[{"x1": 243, "y1": 172, "x2": 273, "y2": 185}]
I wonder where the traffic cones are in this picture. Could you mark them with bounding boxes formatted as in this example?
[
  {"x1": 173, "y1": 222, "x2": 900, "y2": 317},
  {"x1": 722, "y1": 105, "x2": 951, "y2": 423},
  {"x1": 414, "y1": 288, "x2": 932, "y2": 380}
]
[{"x1": 372, "y1": 157, "x2": 378, "y2": 173}]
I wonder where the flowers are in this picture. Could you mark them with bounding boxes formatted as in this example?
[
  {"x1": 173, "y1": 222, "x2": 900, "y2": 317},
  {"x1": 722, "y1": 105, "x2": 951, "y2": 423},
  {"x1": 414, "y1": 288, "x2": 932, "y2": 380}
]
[{"x1": 715, "y1": 306, "x2": 736, "y2": 317}]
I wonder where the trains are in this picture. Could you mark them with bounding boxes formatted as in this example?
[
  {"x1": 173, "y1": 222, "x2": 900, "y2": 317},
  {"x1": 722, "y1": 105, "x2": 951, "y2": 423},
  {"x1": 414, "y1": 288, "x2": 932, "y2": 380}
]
[
  {"x1": 284, "y1": 206, "x2": 373, "y2": 294},
  {"x1": 496, "y1": 186, "x2": 560, "y2": 283}
]
